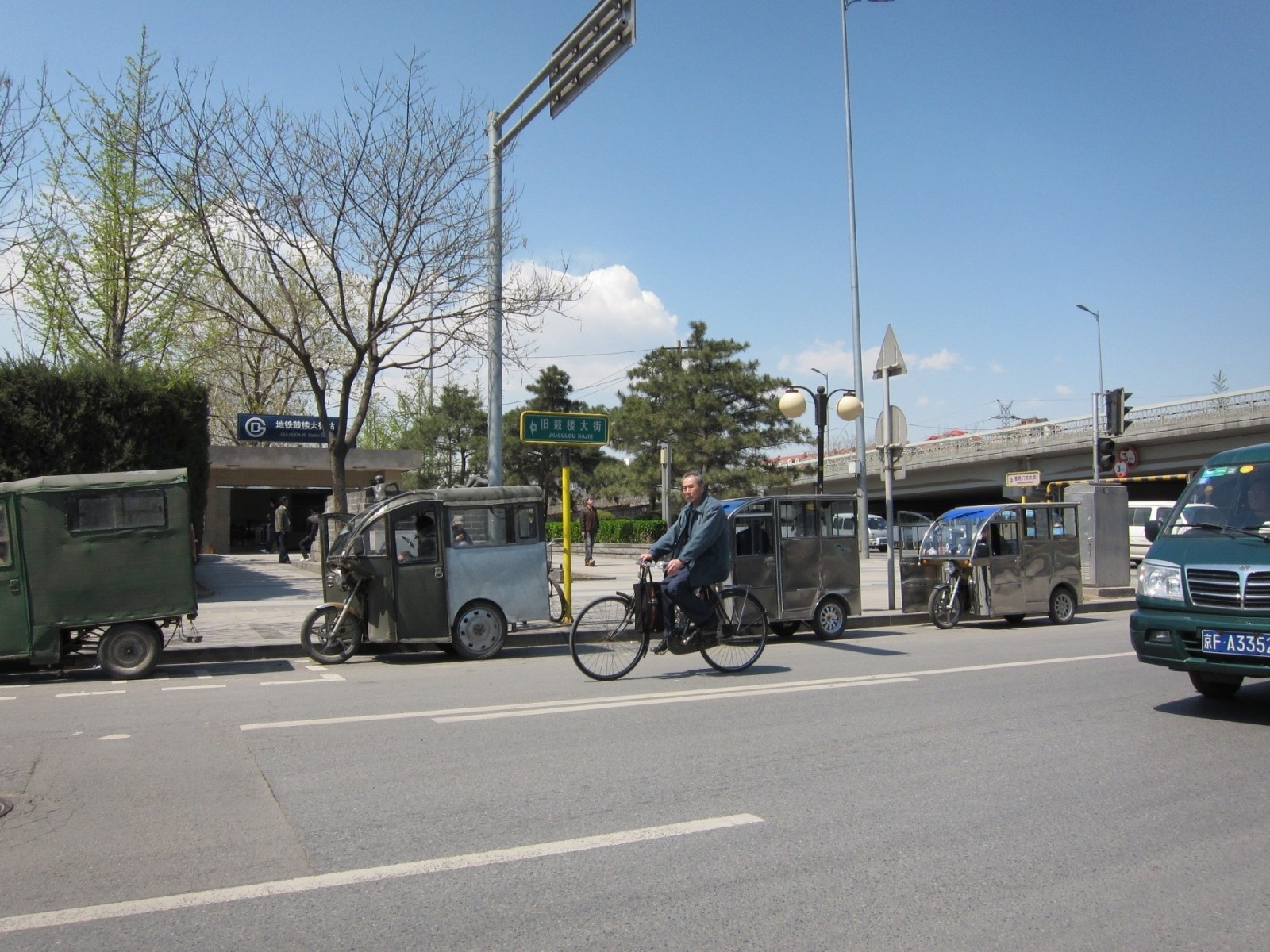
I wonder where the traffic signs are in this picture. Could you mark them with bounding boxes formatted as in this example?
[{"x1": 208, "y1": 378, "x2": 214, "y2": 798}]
[{"x1": 520, "y1": 412, "x2": 610, "y2": 447}]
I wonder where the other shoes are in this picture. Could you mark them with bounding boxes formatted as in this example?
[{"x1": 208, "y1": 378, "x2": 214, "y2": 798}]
[
  {"x1": 261, "y1": 549, "x2": 269, "y2": 553},
  {"x1": 280, "y1": 559, "x2": 292, "y2": 564},
  {"x1": 681, "y1": 629, "x2": 703, "y2": 646},
  {"x1": 301, "y1": 557, "x2": 309, "y2": 562},
  {"x1": 274, "y1": 551, "x2": 278, "y2": 553},
  {"x1": 588, "y1": 559, "x2": 595, "y2": 566},
  {"x1": 650, "y1": 637, "x2": 668, "y2": 653},
  {"x1": 585, "y1": 560, "x2": 588, "y2": 566}
]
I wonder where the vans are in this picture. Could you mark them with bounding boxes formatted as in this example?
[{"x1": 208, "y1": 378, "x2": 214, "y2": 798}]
[
  {"x1": 1000, "y1": 510, "x2": 1062, "y2": 536},
  {"x1": 1129, "y1": 441, "x2": 1270, "y2": 697},
  {"x1": 1128, "y1": 501, "x2": 1217, "y2": 565},
  {"x1": 832, "y1": 512, "x2": 888, "y2": 552}
]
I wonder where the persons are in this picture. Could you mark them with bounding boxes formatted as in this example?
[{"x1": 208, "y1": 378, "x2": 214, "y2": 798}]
[
  {"x1": 975, "y1": 520, "x2": 1014, "y2": 557},
  {"x1": 449, "y1": 515, "x2": 475, "y2": 548},
  {"x1": 455, "y1": 473, "x2": 488, "y2": 489},
  {"x1": 637, "y1": 471, "x2": 730, "y2": 652},
  {"x1": 396, "y1": 515, "x2": 439, "y2": 562},
  {"x1": 579, "y1": 497, "x2": 600, "y2": 566},
  {"x1": 735, "y1": 509, "x2": 775, "y2": 556},
  {"x1": 789, "y1": 503, "x2": 826, "y2": 537},
  {"x1": 259, "y1": 495, "x2": 320, "y2": 561},
  {"x1": 362, "y1": 475, "x2": 399, "y2": 503},
  {"x1": 1221, "y1": 479, "x2": 1270, "y2": 536}
]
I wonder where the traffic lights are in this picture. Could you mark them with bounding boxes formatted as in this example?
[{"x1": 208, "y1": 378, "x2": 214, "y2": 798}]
[
  {"x1": 1117, "y1": 388, "x2": 1133, "y2": 433},
  {"x1": 1097, "y1": 437, "x2": 1116, "y2": 472}
]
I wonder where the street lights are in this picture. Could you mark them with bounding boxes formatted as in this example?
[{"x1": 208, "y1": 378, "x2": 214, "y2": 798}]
[
  {"x1": 1076, "y1": 303, "x2": 1107, "y2": 432},
  {"x1": 778, "y1": 384, "x2": 862, "y2": 492},
  {"x1": 426, "y1": 274, "x2": 468, "y2": 474},
  {"x1": 811, "y1": 368, "x2": 829, "y2": 473}
]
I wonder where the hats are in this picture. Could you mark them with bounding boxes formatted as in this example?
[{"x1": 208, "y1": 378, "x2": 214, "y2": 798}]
[{"x1": 452, "y1": 515, "x2": 464, "y2": 527}]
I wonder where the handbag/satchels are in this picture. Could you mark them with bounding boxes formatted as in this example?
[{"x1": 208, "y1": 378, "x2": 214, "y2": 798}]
[{"x1": 633, "y1": 563, "x2": 664, "y2": 634}]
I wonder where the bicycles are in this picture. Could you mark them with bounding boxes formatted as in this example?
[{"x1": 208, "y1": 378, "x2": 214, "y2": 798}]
[
  {"x1": 569, "y1": 557, "x2": 768, "y2": 682},
  {"x1": 543, "y1": 538, "x2": 566, "y2": 622}
]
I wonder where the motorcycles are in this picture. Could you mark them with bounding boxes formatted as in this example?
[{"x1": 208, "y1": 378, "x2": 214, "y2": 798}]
[
  {"x1": 300, "y1": 484, "x2": 550, "y2": 664},
  {"x1": 673, "y1": 495, "x2": 862, "y2": 640},
  {"x1": 0, "y1": 468, "x2": 202, "y2": 680},
  {"x1": 898, "y1": 502, "x2": 1084, "y2": 629}
]
